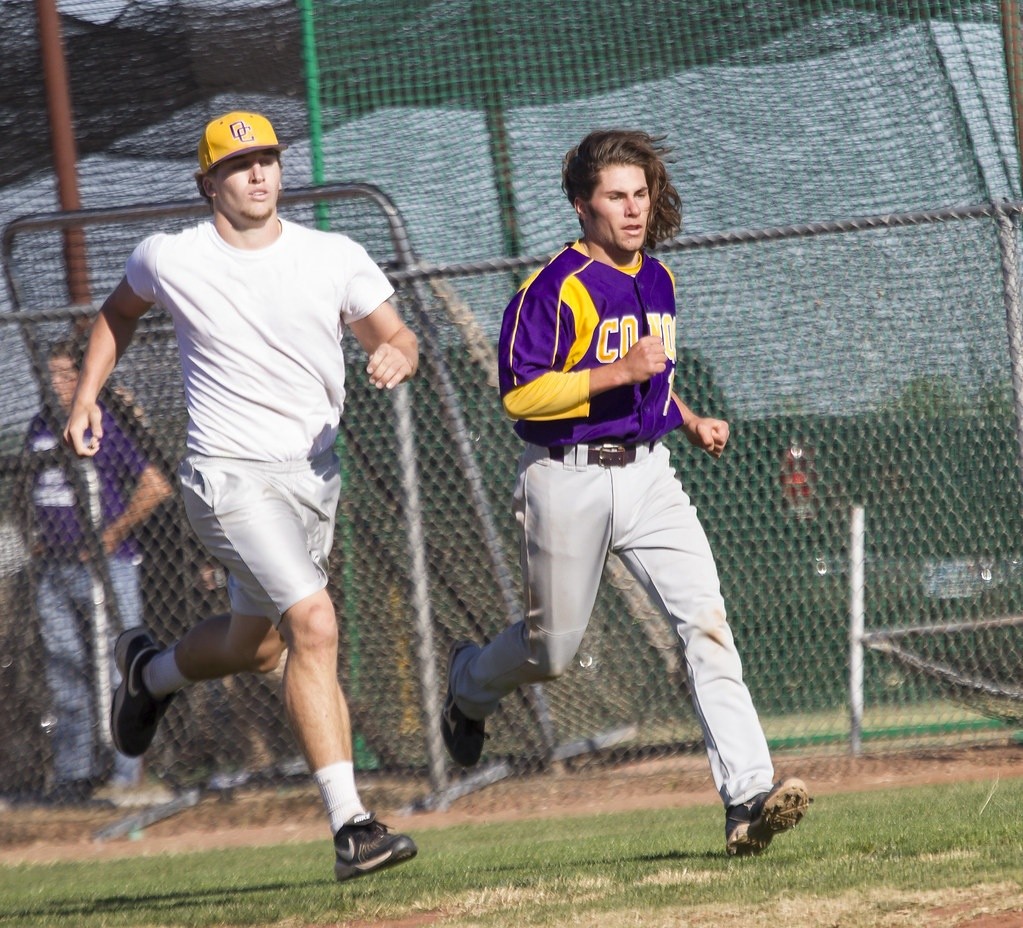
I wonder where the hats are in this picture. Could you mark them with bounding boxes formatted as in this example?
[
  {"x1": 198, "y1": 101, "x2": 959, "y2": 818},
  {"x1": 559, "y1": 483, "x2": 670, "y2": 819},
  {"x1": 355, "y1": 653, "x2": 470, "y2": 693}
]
[{"x1": 197, "y1": 111, "x2": 288, "y2": 175}]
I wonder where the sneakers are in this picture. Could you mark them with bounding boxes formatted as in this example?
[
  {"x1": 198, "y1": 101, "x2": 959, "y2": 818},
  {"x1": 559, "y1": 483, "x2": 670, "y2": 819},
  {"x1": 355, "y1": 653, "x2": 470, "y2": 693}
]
[
  {"x1": 110, "y1": 628, "x2": 183, "y2": 758},
  {"x1": 440, "y1": 639, "x2": 485, "y2": 767},
  {"x1": 725, "y1": 776, "x2": 808, "y2": 857},
  {"x1": 333, "y1": 809, "x2": 416, "y2": 880}
]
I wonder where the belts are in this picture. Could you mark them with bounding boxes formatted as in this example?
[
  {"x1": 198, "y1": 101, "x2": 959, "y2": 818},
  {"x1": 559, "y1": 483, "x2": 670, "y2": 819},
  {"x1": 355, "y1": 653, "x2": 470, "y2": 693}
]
[{"x1": 548, "y1": 442, "x2": 657, "y2": 469}]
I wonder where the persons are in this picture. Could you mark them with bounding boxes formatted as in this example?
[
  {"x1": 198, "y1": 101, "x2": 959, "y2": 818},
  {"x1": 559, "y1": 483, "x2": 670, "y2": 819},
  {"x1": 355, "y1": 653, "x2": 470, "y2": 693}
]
[
  {"x1": 11, "y1": 342, "x2": 171, "y2": 803},
  {"x1": 440, "y1": 129, "x2": 810, "y2": 857},
  {"x1": 136, "y1": 512, "x2": 311, "y2": 789},
  {"x1": 60, "y1": 112, "x2": 419, "y2": 880}
]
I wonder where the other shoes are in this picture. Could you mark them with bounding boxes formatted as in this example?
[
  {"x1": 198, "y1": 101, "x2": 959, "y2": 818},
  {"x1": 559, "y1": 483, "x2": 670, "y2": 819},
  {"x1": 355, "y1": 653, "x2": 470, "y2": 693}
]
[
  {"x1": 39, "y1": 777, "x2": 105, "y2": 809},
  {"x1": 277, "y1": 757, "x2": 311, "y2": 776},
  {"x1": 206, "y1": 762, "x2": 275, "y2": 793}
]
